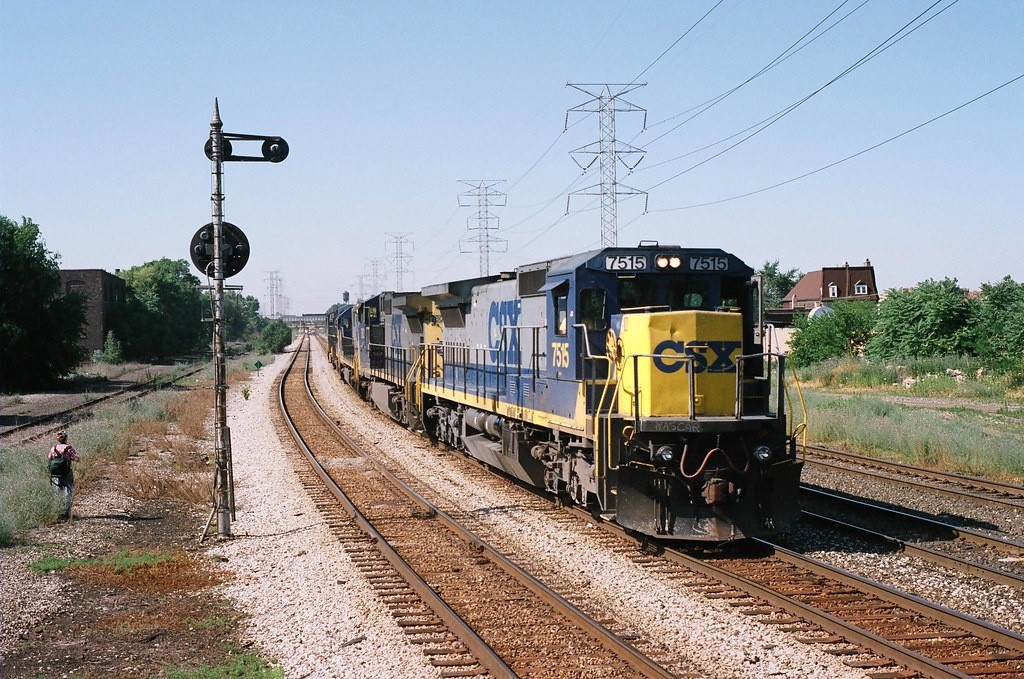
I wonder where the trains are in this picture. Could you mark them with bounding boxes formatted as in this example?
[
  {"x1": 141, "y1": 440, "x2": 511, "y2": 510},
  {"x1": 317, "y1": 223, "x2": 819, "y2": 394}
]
[{"x1": 324, "y1": 239, "x2": 809, "y2": 544}]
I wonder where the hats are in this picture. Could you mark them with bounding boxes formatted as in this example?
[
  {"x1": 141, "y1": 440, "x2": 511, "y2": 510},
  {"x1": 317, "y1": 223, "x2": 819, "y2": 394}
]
[{"x1": 56, "y1": 430, "x2": 67, "y2": 441}]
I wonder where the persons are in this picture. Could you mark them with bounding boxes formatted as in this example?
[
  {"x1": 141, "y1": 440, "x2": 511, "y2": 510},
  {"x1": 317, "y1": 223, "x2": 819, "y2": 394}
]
[{"x1": 47, "y1": 430, "x2": 81, "y2": 522}]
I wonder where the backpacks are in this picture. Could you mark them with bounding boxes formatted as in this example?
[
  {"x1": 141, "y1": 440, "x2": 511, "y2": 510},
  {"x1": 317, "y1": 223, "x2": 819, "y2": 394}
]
[{"x1": 47, "y1": 445, "x2": 72, "y2": 478}]
